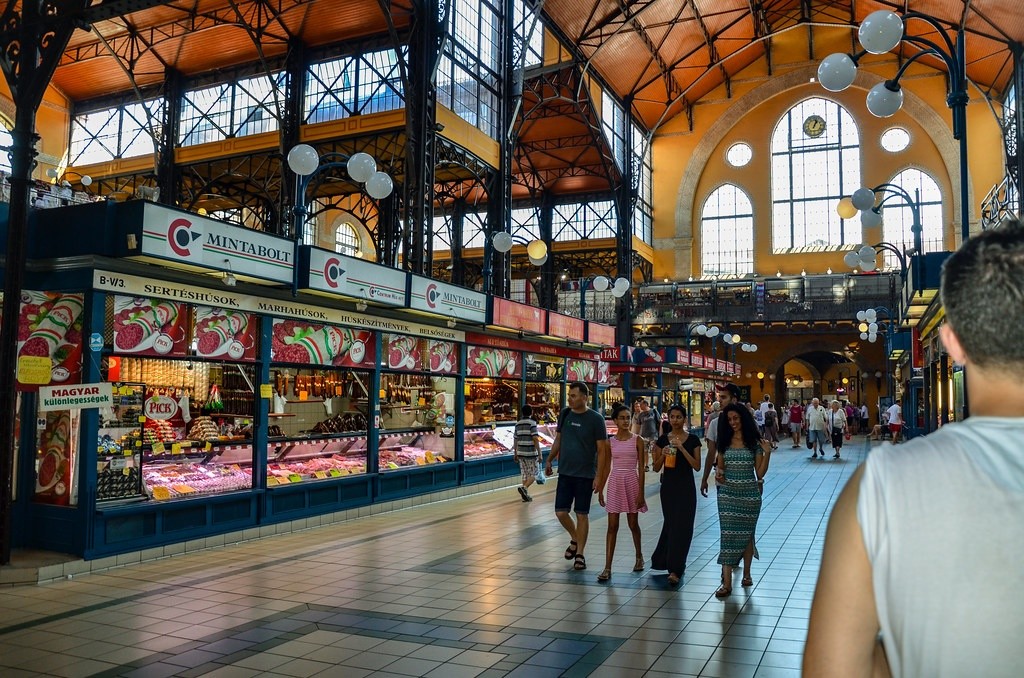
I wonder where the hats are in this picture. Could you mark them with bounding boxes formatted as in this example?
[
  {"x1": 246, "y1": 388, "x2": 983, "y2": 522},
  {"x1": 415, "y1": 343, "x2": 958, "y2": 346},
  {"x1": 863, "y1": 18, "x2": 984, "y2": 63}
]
[{"x1": 714, "y1": 383, "x2": 742, "y2": 401}]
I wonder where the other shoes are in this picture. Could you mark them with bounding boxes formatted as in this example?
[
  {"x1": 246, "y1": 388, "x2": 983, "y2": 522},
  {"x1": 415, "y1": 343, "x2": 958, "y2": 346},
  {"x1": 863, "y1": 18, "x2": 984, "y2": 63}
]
[
  {"x1": 812, "y1": 453, "x2": 817, "y2": 457},
  {"x1": 644, "y1": 466, "x2": 650, "y2": 472},
  {"x1": 774, "y1": 446, "x2": 779, "y2": 450},
  {"x1": 667, "y1": 572, "x2": 679, "y2": 584},
  {"x1": 819, "y1": 449, "x2": 824, "y2": 455},
  {"x1": 792, "y1": 445, "x2": 801, "y2": 448}
]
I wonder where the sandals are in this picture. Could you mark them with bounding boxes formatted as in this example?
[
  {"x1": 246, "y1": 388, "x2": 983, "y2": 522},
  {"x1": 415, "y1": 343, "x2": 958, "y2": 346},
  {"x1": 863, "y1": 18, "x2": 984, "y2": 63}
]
[
  {"x1": 742, "y1": 576, "x2": 752, "y2": 586},
  {"x1": 574, "y1": 554, "x2": 586, "y2": 570},
  {"x1": 716, "y1": 586, "x2": 732, "y2": 597},
  {"x1": 565, "y1": 540, "x2": 577, "y2": 560},
  {"x1": 634, "y1": 555, "x2": 645, "y2": 571},
  {"x1": 598, "y1": 568, "x2": 611, "y2": 580}
]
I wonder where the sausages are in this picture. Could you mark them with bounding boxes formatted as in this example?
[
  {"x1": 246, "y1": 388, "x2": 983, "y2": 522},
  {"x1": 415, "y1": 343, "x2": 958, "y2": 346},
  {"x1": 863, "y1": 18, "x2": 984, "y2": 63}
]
[
  {"x1": 19, "y1": 294, "x2": 85, "y2": 359},
  {"x1": 272, "y1": 369, "x2": 431, "y2": 405},
  {"x1": 112, "y1": 304, "x2": 519, "y2": 377},
  {"x1": 219, "y1": 365, "x2": 256, "y2": 416},
  {"x1": 39, "y1": 409, "x2": 70, "y2": 505},
  {"x1": 469, "y1": 383, "x2": 515, "y2": 415},
  {"x1": 569, "y1": 360, "x2": 611, "y2": 382}
]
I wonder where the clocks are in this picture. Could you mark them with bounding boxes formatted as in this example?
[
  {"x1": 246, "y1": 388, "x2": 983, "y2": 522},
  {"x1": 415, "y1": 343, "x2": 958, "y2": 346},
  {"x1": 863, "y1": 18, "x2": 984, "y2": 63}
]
[{"x1": 804, "y1": 115, "x2": 826, "y2": 138}]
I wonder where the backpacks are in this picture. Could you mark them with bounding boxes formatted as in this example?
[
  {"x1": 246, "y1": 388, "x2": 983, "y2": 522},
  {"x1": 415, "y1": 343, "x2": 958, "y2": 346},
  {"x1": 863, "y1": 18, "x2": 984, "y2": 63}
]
[{"x1": 765, "y1": 411, "x2": 774, "y2": 427}]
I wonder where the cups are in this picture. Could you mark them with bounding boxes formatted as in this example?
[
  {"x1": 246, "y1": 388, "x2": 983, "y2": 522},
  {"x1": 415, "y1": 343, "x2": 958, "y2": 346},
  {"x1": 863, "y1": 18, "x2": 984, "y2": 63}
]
[{"x1": 665, "y1": 445, "x2": 677, "y2": 468}]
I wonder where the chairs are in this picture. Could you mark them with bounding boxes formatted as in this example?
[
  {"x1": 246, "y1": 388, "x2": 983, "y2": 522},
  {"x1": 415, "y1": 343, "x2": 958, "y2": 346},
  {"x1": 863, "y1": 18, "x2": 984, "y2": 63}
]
[{"x1": 880, "y1": 422, "x2": 903, "y2": 441}]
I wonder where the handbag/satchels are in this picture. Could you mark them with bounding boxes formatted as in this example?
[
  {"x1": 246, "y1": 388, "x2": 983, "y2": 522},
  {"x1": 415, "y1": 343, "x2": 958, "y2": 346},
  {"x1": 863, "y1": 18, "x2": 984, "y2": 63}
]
[
  {"x1": 535, "y1": 460, "x2": 546, "y2": 485},
  {"x1": 806, "y1": 432, "x2": 813, "y2": 449},
  {"x1": 832, "y1": 427, "x2": 841, "y2": 437},
  {"x1": 845, "y1": 432, "x2": 852, "y2": 440}
]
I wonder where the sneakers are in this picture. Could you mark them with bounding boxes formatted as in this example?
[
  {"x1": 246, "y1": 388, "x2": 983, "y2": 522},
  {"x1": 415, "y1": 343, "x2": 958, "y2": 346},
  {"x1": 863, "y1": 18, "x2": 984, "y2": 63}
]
[{"x1": 518, "y1": 486, "x2": 532, "y2": 502}]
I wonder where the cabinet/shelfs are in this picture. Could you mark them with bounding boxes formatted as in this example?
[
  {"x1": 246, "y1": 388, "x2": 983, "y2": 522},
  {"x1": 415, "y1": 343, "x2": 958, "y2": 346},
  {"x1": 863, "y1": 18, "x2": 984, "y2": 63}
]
[{"x1": 97, "y1": 382, "x2": 143, "y2": 503}]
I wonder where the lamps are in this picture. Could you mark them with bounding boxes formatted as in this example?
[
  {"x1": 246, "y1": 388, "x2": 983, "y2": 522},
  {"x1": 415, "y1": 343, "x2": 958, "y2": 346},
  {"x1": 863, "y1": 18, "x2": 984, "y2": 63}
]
[
  {"x1": 447, "y1": 308, "x2": 456, "y2": 327},
  {"x1": 356, "y1": 289, "x2": 367, "y2": 311},
  {"x1": 223, "y1": 260, "x2": 236, "y2": 286}
]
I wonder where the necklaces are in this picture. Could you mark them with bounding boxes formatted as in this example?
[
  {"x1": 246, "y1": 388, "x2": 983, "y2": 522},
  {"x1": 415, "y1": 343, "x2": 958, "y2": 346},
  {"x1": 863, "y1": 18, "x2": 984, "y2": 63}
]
[{"x1": 677, "y1": 437, "x2": 678, "y2": 439}]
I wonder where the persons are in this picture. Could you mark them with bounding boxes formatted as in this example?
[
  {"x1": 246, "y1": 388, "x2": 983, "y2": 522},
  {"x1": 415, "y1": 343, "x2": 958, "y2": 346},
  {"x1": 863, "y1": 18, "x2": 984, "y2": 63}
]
[
  {"x1": 867, "y1": 400, "x2": 906, "y2": 443},
  {"x1": 802, "y1": 217, "x2": 1024, "y2": 677},
  {"x1": 806, "y1": 398, "x2": 848, "y2": 458},
  {"x1": 650, "y1": 406, "x2": 702, "y2": 583},
  {"x1": 822, "y1": 399, "x2": 869, "y2": 434},
  {"x1": 597, "y1": 403, "x2": 648, "y2": 579},
  {"x1": 747, "y1": 394, "x2": 810, "y2": 450},
  {"x1": 545, "y1": 382, "x2": 608, "y2": 570},
  {"x1": 700, "y1": 383, "x2": 771, "y2": 595},
  {"x1": 514, "y1": 405, "x2": 542, "y2": 502},
  {"x1": 464, "y1": 395, "x2": 474, "y2": 423},
  {"x1": 704, "y1": 402, "x2": 723, "y2": 469},
  {"x1": 632, "y1": 397, "x2": 662, "y2": 472}
]
